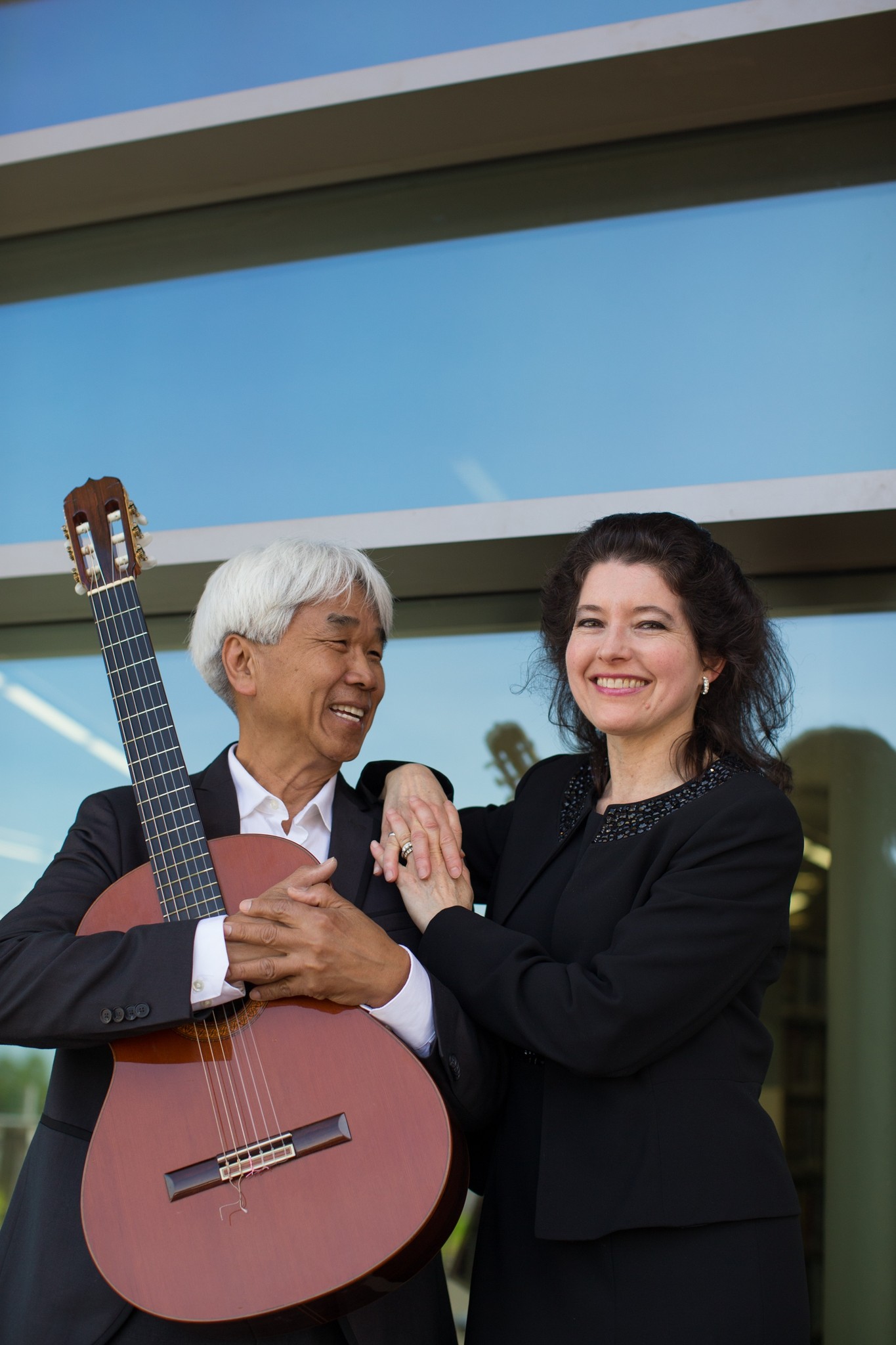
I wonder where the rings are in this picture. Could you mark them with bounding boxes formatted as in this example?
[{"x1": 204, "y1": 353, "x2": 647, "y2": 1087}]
[
  {"x1": 388, "y1": 832, "x2": 395, "y2": 838},
  {"x1": 401, "y1": 841, "x2": 413, "y2": 859}
]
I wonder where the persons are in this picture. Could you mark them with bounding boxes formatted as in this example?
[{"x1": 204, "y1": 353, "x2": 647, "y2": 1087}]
[
  {"x1": 0, "y1": 536, "x2": 460, "y2": 1345},
  {"x1": 355, "y1": 512, "x2": 813, "y2": 1345}
]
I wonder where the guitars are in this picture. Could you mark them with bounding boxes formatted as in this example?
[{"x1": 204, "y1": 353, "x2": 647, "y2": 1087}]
[{"x1": 58, "y1": 473, "x2": 473, "y2": 1327}]
